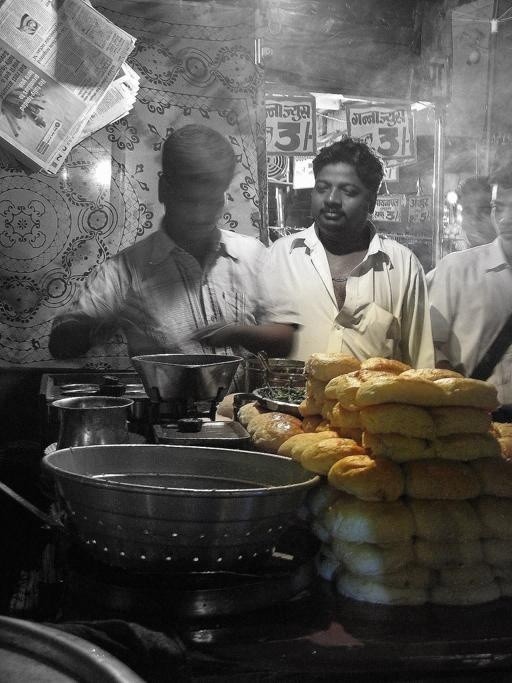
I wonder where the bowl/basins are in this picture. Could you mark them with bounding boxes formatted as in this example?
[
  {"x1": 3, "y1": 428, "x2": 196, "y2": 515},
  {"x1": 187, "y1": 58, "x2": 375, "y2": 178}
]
[
  {"x1": 131, "y1": 351, "x2": 243, "y2": 407},
  {"x1": 246, "y1": 355, "x2": 307, "y2": 389}
]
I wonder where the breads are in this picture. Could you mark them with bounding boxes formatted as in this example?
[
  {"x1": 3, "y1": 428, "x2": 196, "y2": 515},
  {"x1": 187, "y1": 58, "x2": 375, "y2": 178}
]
[{"x1": 236, "y1": 353, "x2": 512, "y2": 606}]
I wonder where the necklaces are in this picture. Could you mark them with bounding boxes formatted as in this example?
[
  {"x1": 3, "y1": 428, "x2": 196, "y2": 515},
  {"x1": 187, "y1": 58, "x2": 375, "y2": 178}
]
[{"x1": 332, "y1": 272, "x2": 349, "y2": 282}]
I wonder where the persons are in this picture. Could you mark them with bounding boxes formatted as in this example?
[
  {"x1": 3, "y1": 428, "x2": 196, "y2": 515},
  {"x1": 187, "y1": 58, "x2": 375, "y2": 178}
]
[
  {"x1": 268, "y1": 140, "x2": 436, "y2": 365},
  {"x1": 423, "y1": 173, "x2": 496, "y2": 291},
  {"x1": 430, "y1": 163, "x2": 512, "y2": 423},
  {"x1": 50, "y1": 125, "x2": 303, "y2": 397}
]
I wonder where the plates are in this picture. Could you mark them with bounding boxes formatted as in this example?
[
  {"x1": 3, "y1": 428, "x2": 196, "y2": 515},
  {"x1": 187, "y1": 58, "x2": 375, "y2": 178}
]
[{"x1": 254, "y1": 386, "x2": 307, "y2": 416}]
